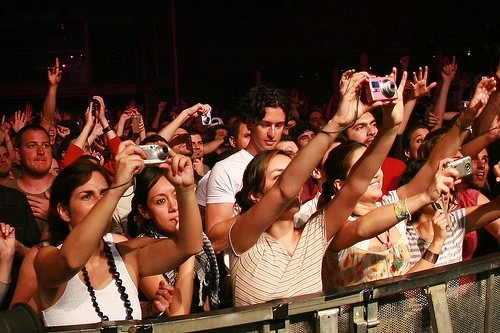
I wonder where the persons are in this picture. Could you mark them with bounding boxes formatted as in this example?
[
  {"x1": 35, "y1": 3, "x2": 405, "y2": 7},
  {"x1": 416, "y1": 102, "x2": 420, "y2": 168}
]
[{"x1": 0, "y1": 52, "x2": 500, "y2": 332}]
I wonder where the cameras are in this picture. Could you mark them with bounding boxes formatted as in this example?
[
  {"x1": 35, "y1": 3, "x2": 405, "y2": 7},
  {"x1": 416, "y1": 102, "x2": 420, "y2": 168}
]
[
  {"x1": 364, "y1": 77, "x2": 399, "y2": 102},
  {"x1": 136, "y1": 145, "x2": 170, "y2": 164},
  {"x1": 445, "y1": 156, "x2": 473, "y2": 180},
  {"x1": 201, "y1": 109, "x2": 212, "y2": 126}
]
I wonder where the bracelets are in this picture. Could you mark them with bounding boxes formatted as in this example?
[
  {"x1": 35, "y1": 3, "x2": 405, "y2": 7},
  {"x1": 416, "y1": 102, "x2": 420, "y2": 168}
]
[
  {"x1": 422, "y1": 248, "x2": 440, "y2": 265},
  {"x1": 457, "y1": 118, "x2": 473, "y2": 134},
  {"x1": 392, "y1": 197, "x2": 412, "y2": 223},
  {"x1": 101, "y1": 125, "x2": 114, "y2": 134}
]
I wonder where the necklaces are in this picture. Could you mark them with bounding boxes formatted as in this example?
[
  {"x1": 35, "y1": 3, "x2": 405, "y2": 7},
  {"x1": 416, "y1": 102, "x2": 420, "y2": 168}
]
[
  {"x1": 375, "y1": 229, "x2": 391, "y2": 252},
  {"x1": 80, "y1": 242, "x2": 134, "y2": 322}
]
